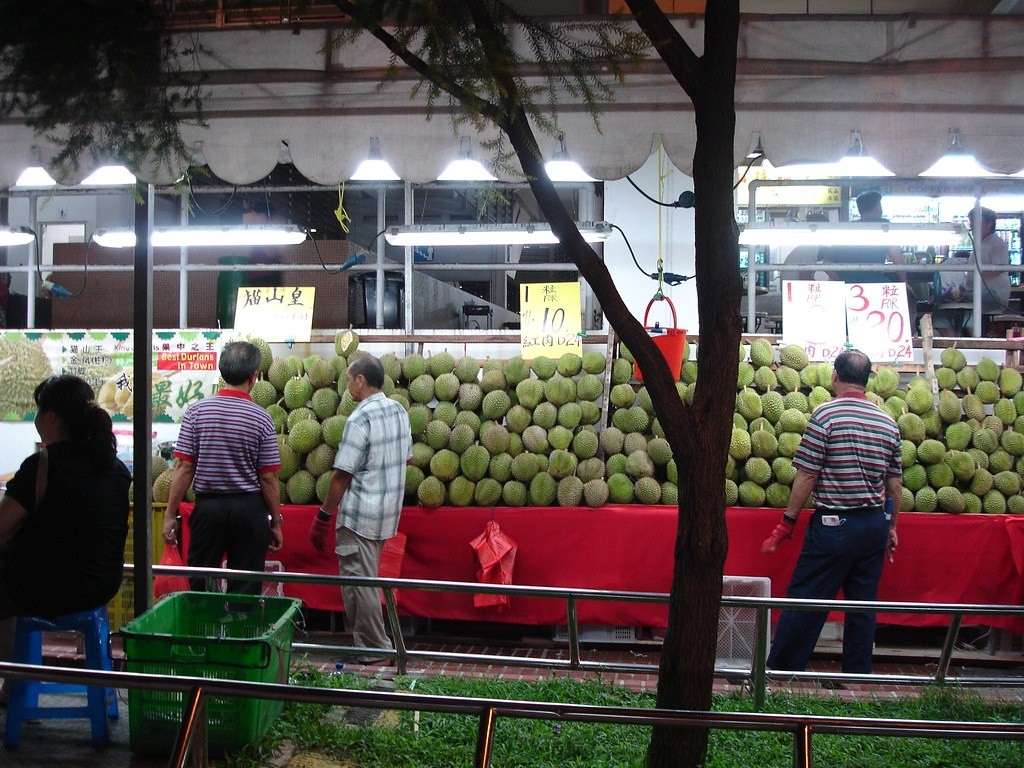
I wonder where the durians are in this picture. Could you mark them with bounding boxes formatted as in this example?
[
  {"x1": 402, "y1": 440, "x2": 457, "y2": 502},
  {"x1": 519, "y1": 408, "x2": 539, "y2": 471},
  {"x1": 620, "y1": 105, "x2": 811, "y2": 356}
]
[
  {"x1": 866, "y1": 340, "x2": 1024, "y2": 514},
  {"x1": 129, "y1": 455, "x2": 199, "y2": 504},
  {"x1": 378, "y1": 333, "x2": 696, "y2": 507},
  {"x1": 725, "y1": 338, "x2": 836, "y2": 509},
  {"x1": 217, "y1": 322, "x2": 373, "y2": 507},
  {"x1": 0, "y1": 335, "x2": 171, "y2": 423}
]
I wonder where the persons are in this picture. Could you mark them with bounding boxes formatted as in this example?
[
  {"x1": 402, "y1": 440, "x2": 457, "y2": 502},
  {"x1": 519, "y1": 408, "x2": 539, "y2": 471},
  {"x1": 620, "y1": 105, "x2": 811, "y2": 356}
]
[
  {"x1": 778, "y1": 213, "x2": 829, "y2": 292},
  {"x1": 161, "y1": 341, "x2": 284, "y2": 613},
  {"x1": 824, "y1": 190, "x2": 918, "y2": 338},
  {"x1": 0, "y1": 374, "x2": 132, "y2": 722},
  {"x1": 308, "y1": 355, "x2": 413, "y2": 668},
  {"x1": 727, "y1": 349, "x2": 902, "y2": 687},
  {"x1": 931, "y1": 206, "x2": 1011, "y2": 337}
]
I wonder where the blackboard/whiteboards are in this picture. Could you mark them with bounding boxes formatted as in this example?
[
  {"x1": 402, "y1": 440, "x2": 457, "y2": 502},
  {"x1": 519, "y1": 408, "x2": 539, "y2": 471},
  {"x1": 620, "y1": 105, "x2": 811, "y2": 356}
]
[
  {"x1": 782, "y1": 278, "x2": 847, "y2": 362},
  {"x1": 843, "y1": 282, "x2": 914, "y2": 362}
]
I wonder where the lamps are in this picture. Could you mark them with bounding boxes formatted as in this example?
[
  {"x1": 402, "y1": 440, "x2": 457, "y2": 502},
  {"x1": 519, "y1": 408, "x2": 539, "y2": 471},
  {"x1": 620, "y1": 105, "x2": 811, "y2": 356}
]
[
  {"x1": 16, "y1": 144, "x2": 137, "y2": 187},
  {"x1": 345, "y1": 129, "x2": 604, "y2": 182},
  {"x1": 383, "y1": 221, "x2": 613, "y2": 245},
  {"x1": 744, "y1": 126, "x2": 1000, "y2": 178},
  {"x1": 93, "y1": 225, "x2": 307, "y2": 248}
]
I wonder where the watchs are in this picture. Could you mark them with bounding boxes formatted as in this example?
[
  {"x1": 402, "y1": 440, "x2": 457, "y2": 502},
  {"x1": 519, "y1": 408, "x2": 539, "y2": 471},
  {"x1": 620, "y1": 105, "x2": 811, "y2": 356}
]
[{"x1": 272, "y1": 514, "x2": 284, "y2": 523}]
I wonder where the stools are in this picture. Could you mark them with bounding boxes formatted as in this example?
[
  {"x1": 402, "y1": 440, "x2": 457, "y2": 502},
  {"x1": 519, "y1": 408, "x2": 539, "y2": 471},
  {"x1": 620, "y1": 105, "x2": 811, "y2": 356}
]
[
  {"x1": 937, "y1": 302, "x2": 977, "y2": 338},
  {"x1": 739, "y1": 311, "x2": 782, "y2": 333},
  {"x1": 5, "y1": 604, "x2": 118, "y2": 748},
  {"x1": 988, "y1": 313, "x2": 1022, "y2": 340}
]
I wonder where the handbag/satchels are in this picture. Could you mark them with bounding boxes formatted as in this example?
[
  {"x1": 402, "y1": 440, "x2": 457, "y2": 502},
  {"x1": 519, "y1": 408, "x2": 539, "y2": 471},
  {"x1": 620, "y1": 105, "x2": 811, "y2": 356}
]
[{"x1": 634, "y1": 296, "x2": 688, "y2": 382}]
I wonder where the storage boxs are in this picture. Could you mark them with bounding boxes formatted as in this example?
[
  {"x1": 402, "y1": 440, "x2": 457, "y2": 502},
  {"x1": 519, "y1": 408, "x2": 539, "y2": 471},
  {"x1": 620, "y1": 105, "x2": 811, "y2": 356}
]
[{"x1": 118, "y1": 591, "x2": 303, "y2": 755}]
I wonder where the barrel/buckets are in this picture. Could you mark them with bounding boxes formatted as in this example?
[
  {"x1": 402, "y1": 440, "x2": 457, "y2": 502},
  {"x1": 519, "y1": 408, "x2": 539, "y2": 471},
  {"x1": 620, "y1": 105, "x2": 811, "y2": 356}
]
[{"x1": 633, "y1": 296, "x2": 689, "y2": 381}]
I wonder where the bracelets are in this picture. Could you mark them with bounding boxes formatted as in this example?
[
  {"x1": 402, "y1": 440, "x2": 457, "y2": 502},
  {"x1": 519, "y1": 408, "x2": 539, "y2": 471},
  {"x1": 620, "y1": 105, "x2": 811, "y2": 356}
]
[
  {"x1": 317, "y1": 508, "x2": 332, "y2": 522},
  {"x1": 783, "y1": 513, "x2": 796, "y2": 522}
]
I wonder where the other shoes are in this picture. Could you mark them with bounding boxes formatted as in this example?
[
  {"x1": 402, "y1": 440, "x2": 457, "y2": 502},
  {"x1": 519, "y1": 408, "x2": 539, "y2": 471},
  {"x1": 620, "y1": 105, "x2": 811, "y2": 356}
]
[
  {"x1": 821, "y1": 682, "x2": 847, "y2": 690},
  {"x1": 343, "y1": 657, "x2": 387, "y2": 666},
  {"x1": 727, "y1": 676, "x2": 768, "y2": 686}
]
